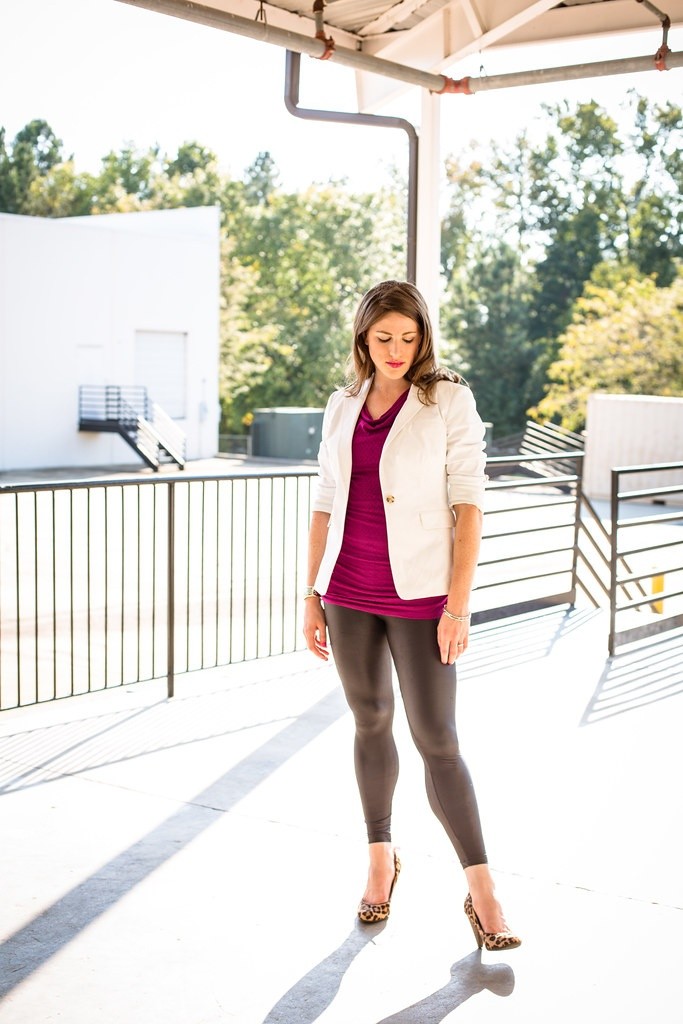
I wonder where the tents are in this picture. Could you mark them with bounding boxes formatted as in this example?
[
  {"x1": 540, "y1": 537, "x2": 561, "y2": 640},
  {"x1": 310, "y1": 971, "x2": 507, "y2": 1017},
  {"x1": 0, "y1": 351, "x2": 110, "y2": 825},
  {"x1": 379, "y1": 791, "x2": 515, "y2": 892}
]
[{"x1": 112, "y1": 0, "x2": 683, "y2": 371}]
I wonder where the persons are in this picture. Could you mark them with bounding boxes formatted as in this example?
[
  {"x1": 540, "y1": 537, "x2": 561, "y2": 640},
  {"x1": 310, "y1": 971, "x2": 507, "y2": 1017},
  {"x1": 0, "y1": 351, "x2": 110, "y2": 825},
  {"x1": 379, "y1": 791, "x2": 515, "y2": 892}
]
[{"x1": 303, "y1": 284, "x2": 522, "y2": 952}]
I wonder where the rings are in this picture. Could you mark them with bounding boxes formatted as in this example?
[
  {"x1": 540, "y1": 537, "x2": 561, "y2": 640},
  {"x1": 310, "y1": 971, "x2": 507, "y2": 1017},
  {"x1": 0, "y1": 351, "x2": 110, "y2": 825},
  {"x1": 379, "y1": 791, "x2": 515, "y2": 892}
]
[{"x1": 457, "y1": 643, "x2": 464, "y2": 647}]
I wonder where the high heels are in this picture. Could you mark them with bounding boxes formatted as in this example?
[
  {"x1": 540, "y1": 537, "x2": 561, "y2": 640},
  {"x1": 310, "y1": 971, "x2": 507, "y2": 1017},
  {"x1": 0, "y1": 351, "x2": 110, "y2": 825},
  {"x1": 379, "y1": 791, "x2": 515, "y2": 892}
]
[
  {"x1": 463, "y1": 893, "x2": 521, "y2": 953},
  {"x1": 358, "y1": 851, "x2": 400, "y2": 922}
]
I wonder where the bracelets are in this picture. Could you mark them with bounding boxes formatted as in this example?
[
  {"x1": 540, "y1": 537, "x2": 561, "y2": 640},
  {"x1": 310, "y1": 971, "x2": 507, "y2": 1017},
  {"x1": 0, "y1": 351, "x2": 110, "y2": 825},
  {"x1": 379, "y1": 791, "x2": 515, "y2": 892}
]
[
  {"x1": 443, "y1": 604, "x2": 471, "y2": 622},
  {"x1": 304, "y1": 585, "x2": 319, "y2": 600}
]
